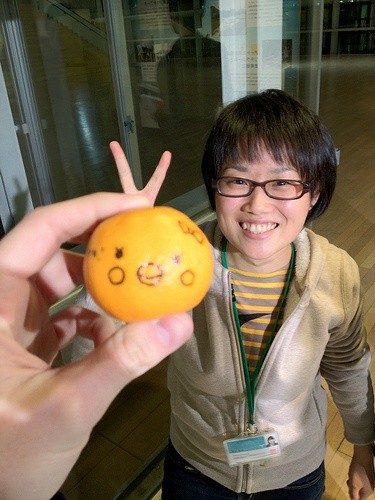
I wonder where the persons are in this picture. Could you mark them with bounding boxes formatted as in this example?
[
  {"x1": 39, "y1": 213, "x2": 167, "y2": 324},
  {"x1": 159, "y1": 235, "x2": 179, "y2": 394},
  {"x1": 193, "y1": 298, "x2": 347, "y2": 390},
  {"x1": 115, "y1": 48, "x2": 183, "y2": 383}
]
[
  {"x1": 36, "y1": 0, "x2": 223, "y2": 167},
  {"x1": 264, "y1": 435, "x2": 278, "y2": 448},
  {"x1": 0, "y1": 191, "x2": 196, "y2": 499},
  {"x1": 108, "y1": 89, "x2": 375, "y2": 500}
]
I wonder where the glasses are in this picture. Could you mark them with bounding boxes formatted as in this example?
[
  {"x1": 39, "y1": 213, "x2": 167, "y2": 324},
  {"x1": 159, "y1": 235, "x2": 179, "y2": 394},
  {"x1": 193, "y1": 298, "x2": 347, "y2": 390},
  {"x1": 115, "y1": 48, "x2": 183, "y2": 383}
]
[{"x1": 212, "y1": 176, "x2": 312, "y2": 200}]
